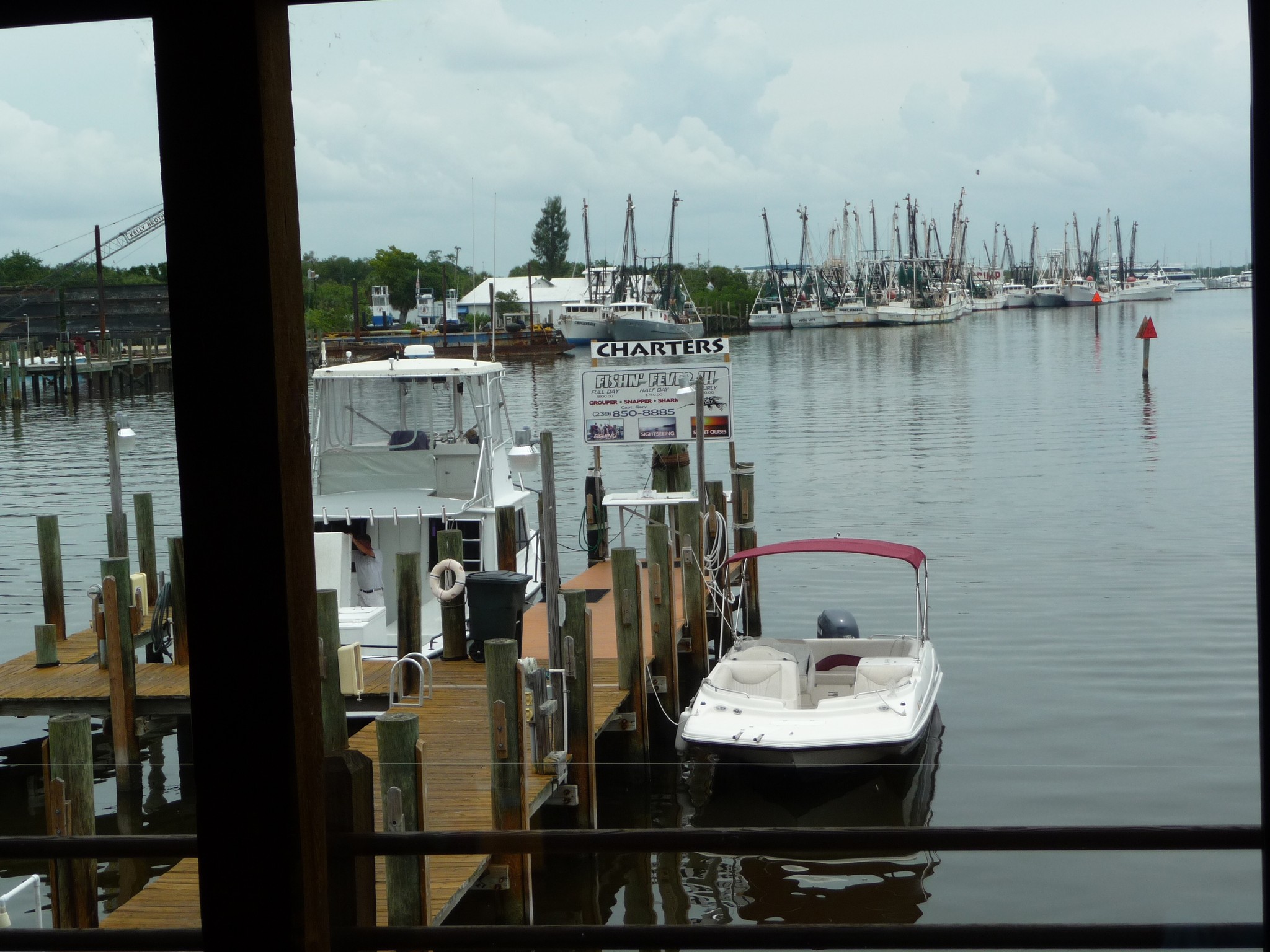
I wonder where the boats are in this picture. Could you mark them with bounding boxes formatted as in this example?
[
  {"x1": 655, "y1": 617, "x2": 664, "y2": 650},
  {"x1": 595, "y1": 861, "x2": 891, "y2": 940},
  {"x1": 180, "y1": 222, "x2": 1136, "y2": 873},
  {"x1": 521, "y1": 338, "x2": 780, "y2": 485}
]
[
  {"x1": 0, "y1": 355, "x2": 86, "y2": 379},
  {"x1": 746, "y1": 183, "x2": 1251, "y2": 337},
  {"x1": 668, "y1": 532, "x2": 946, "y2": 776},
  {"x1": 316, "y1": 191, "x2": 706, "y2": 362},
  {"x1": 305, "y1": 354, "x2": 545, "y2": 727}
]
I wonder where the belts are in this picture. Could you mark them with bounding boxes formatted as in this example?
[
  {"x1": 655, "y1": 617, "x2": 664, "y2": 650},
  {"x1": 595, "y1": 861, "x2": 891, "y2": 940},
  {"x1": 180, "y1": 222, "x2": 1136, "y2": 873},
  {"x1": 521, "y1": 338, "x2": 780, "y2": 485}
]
[{"x1": 360, "y1": 587, "x2": 382, "y2": 593}]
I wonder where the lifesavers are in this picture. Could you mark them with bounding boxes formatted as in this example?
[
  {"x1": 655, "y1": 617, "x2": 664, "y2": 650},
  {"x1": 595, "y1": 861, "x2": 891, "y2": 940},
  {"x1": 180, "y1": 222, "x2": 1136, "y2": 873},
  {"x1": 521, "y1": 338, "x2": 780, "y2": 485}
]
[
  {"x1": 944, "y1": 295, "x2": 947, "y2": 300},
  {"x1": 664, "y1": 314, "x2": 667, "y2": 320},
  {"x1": 429, "y1": 559, "x2": 466, "y2": 600},
  {"x1": 1126, "y1": 285, "x2": 1129, "y2": 288}
]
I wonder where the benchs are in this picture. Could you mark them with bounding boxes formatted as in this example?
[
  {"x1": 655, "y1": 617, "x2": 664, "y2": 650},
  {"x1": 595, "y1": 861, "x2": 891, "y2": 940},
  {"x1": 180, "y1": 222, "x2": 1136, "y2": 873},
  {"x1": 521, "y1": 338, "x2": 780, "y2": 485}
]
[{"x1": 806, "y1": 638, "x2": 916, "y2": 684}]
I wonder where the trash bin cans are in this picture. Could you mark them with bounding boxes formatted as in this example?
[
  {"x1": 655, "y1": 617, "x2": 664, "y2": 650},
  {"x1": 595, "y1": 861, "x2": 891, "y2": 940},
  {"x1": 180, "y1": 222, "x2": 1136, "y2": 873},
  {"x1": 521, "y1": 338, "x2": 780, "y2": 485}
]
[{"x1": 464, "y1": 570, "x2": 532, "y2": 660}]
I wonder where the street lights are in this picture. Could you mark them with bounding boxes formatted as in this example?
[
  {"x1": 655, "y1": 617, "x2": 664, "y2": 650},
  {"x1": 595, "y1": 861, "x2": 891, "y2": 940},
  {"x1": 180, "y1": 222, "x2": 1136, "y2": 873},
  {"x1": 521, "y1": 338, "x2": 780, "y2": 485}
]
[{"x1": 23, "y1": 314, "x2": 30, "y2": 359}]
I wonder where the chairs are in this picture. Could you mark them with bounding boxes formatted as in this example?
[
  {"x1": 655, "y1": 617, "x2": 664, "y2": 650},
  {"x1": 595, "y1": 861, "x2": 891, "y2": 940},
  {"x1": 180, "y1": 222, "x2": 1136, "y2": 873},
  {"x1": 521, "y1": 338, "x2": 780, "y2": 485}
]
[
  {"x1": 718, "y1": 638, "x2": 915, "y2": 707},
  {"x1": 389, "y1": 430, "x2": 428, "y2": 451}
]
